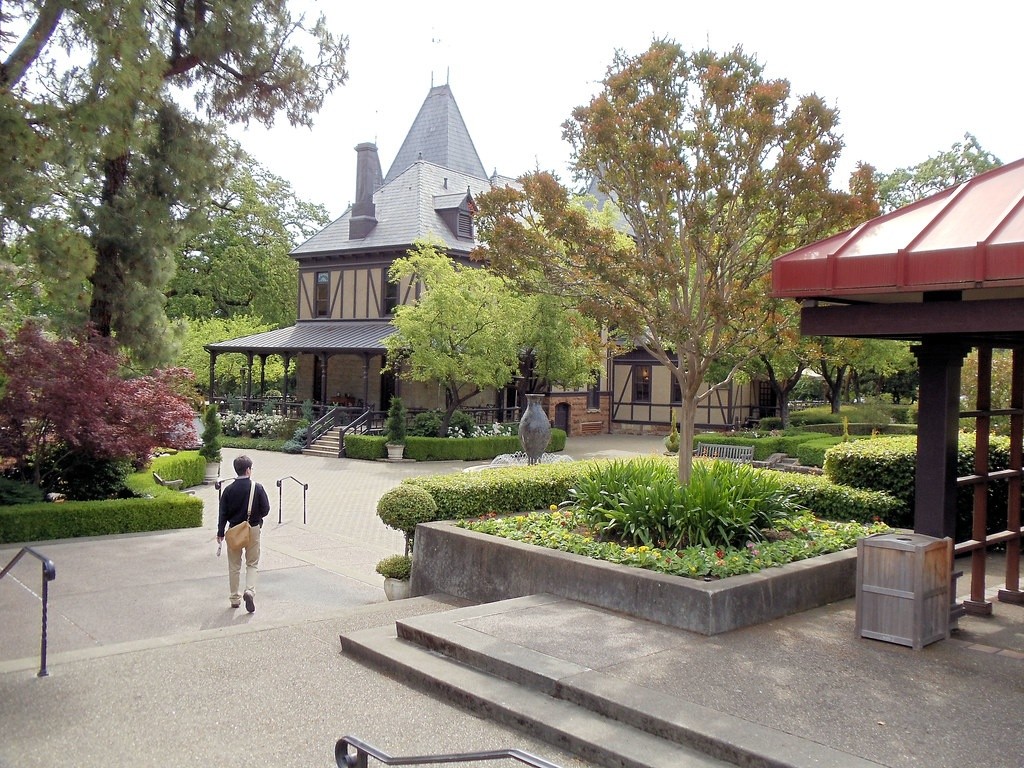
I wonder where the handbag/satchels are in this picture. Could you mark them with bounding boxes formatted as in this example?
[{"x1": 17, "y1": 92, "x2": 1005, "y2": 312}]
[{"x1": 224, "y1": 521, "x2": 251, "y2": 551}]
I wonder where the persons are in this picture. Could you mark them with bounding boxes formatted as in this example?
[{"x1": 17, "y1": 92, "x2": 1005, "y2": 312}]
[{"x1": 216, "y1": 456, "x2": 270, "y2": 612}]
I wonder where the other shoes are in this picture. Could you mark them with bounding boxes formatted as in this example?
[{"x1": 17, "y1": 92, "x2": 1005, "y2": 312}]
[
  {"x1": 231, "y1": 604, "x2": 239, "y2": 608},
  {"x1": 243, "y1": 592, "x2": 255, "y2": 612}
]
